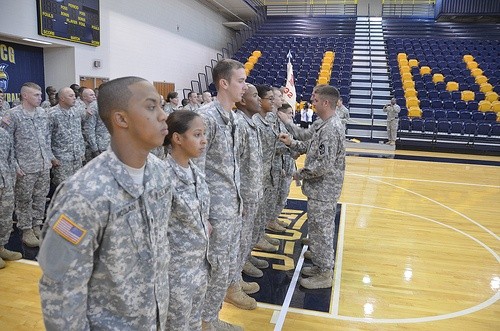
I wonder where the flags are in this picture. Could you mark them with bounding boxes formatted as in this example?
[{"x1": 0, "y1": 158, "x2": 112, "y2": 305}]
[{"x1": 282, "y1": 63, "x2": 296, "y2": 118}]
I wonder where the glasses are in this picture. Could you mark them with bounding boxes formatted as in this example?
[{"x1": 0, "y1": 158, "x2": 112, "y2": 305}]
[{"x1": 264, "y1": 96, "x2": 276, "y2": 104}]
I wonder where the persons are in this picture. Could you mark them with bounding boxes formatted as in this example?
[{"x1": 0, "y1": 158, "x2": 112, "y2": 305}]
[
  {"x1": 0, "y1": 58, "x2": 350, "y2": 331},
  {"x1": 383, "y1": 98, "x2": 402, "y2": 145}
]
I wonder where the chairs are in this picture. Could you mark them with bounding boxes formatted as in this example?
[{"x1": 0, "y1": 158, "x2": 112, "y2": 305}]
[{"x1": 204, "y1": 37, "x2": 500, "y2": 146}]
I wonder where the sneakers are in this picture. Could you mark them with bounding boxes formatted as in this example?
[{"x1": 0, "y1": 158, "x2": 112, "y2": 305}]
[
  {"x1": 224, "y1": 287, "x2": 258, "y2": 310},
  {"x1": 33, "y1": 225, "x2": 41, "y2": 238},
  {"x1": 300, "y1": 235, "x2": 311, "y2": 246},
  {"x1": 0, "y1": 256, "x2": 6, "y2": 268},
  {"x1": 275, "y1": 221, "x2": 291, "y2": 228},
  {"x1": 391, "y1": 141, "x2": 395, "y2": 146},
  {"x1": 298, "y1": 270, "x2": 334, "y2": 290},
  {"x1": 303, "y1": 251, "x2": 312, "y2": 259},
  {"x1": 0, "y1": 246, "x2": 22, "y2": 261},
  {"x1": 265, "y1": 235, "x2": 280, "y2": 246},
  {"x1": 22, "y1": 228, "x2": 40, "y2": 246},
  {"x1": 302, "y1": 267, "x2": 319, "y2": 275},
  {"x1": 236, "y1": 280, "x2": 260, "y2": 294},
  {"x1": 385, "y1": 140, "x2": 390, "y2": 145},
  {"x1": 247, "y1": 255, "x2": 271, "y2": 269},
  {"x1": 243, "y1": 261, "x2": 266, "y2": 276},
  {"x1": 254, "y1": 237, "x2": 279, "y2": 251},
  {"x1": 266, "y1": 221, "x2": 287, "y2": 232}
]
